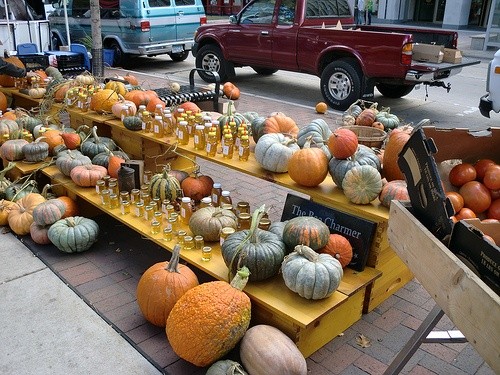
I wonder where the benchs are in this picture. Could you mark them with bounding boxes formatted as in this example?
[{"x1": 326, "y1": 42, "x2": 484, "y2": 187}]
[
  {"x1": 103, "y1": 68, "x2": 219, "y2": 112},
  {"x1": 469, "y1": 33, "x2": 497, "y2": 50}
]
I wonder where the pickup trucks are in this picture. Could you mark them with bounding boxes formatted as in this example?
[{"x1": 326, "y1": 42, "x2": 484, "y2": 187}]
[
  {"x1": 478, "y1": 49, "x2": 500, "y2": 118},
  {"x1": 192, "y1": 0, "x2": 481, "y2": 113}
]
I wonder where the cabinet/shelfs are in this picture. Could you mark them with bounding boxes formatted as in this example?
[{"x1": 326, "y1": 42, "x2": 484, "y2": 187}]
[{"x1": 0, "y1": 82, "x2": 416, "y2": 360}]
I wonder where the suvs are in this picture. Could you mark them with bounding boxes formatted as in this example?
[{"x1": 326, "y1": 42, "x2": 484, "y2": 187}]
[{"x1": 46, "y1": 0, "x2": 207, "y2": 67}]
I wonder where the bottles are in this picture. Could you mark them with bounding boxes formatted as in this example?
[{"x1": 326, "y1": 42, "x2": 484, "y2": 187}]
[
  {"x1": 121, "y1": 103, "x2": 251, "y2": 162},
  {"x1": 13, "y1": 75, "x2": 101, "y2": 112},
  {"x1": 95, "y1": 170, "x2": 272, "y2": 261},
  {"x1": 3, "y1": 128, "x2": 50, "y2": 145}
]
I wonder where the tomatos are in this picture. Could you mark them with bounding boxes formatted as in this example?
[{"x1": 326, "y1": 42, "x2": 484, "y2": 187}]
[{"x1": 438, "y1": 158, "x2": 500, "y2": 248}]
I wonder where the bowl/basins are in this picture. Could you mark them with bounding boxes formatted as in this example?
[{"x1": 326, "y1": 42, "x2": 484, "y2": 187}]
[{"x1": 335, "y1": 125, "x2": 387, "y2": 150}]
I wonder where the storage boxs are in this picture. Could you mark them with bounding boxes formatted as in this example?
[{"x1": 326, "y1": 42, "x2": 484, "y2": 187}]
[
  {"x1": 398, "y1": 126, "x2": 500, "y2": 290},
  {"x1": 412, "y1": 42, "x2": 463, "y2": 64},
  {"x1": 14, "y1": 53, "x2": 87, "y2": 78}
]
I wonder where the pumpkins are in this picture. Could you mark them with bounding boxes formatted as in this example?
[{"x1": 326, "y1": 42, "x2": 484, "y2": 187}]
[
  {"x1": 148, "y1": 164, "x2": 353, "y2": 301},
  {"x1": 137, "y1": 245, "x2": 308, "y2": 375},
  {"x1": 250, "y1": 100, "x2": 430, "y2": 206},
  {"x1": 0, "y1": 91, "x2": 133, "y2": 253},
  {"x1": 0, "y1": 49, "x2": 26, "y2": 87},
  {"x1": 28, "y1": 66, "x2": 259, "y2": 138}
]
